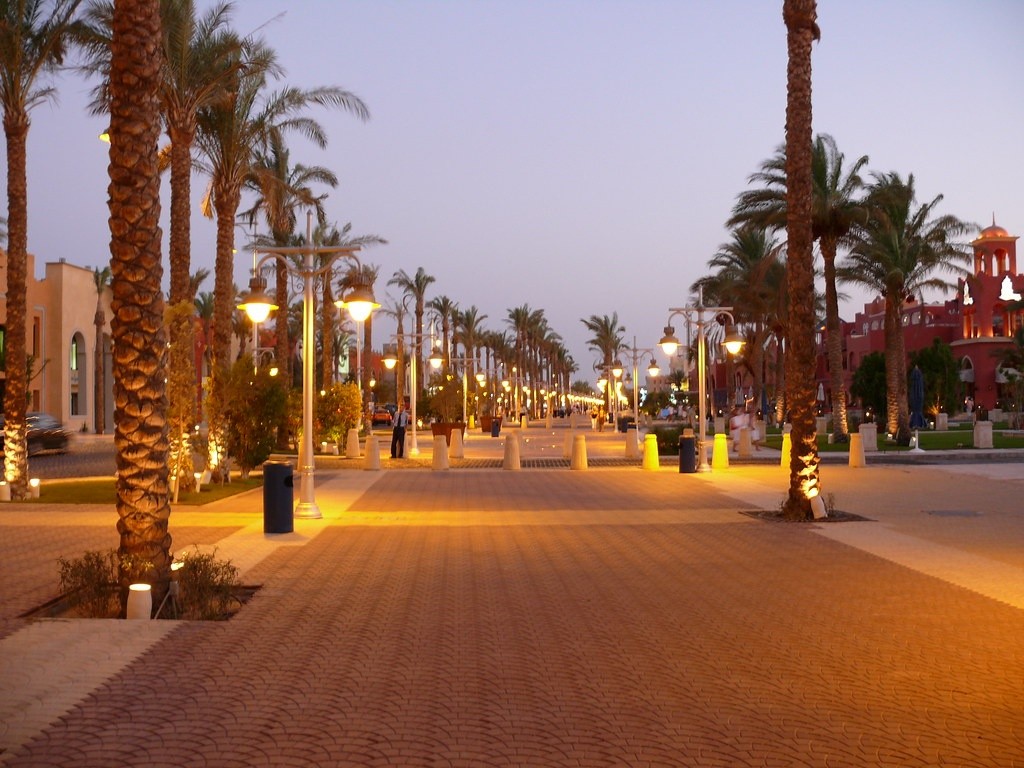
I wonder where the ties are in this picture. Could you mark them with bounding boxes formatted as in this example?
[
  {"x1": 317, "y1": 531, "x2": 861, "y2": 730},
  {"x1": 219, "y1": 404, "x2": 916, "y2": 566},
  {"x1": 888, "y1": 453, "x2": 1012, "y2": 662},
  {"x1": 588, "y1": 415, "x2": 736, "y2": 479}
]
[{"x1": 397, "y1": 413, "x2": 401, "y2": 427}]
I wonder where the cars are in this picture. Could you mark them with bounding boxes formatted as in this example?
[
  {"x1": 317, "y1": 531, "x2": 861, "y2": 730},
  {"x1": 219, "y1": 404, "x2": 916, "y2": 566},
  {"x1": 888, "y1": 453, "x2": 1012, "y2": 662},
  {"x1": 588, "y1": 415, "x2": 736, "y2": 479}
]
[
  {"x1": 0, "y1": 412, "x2": 69, "y2": 460},
  {"x1": 362, "y1": 402, "x2": 412, "y2": 426}
]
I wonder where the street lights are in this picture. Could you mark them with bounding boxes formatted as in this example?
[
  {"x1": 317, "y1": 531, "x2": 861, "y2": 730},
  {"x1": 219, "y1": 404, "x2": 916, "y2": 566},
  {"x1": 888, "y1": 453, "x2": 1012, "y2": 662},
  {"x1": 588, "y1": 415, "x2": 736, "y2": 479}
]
[
  {"x1": 477, "y1": 359, "x2": 634, "y2": 425},
  {"x1": 379, "y1": 315, "x2": 445, "y2": 452},
  {"x1": 235, "y1": 208, "x2": 382, "y2": 520},
  {"x1": 609, "y1": 334, "x2": 662, "y2": 444},
  {"x1": 428, "y1": 347, "x2": 485, "y2": 436},
  {"x1": 657, "y1": 281, "x2": 747, "y2": 470}
]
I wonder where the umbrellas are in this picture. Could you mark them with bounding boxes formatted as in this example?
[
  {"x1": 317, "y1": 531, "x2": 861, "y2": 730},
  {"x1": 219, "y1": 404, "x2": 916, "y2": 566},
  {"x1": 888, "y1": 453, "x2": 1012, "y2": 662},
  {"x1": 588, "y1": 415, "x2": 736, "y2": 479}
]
[
  {"x1": 749, "y1": 387, "x2": 753, "y2": 398},
  {"x1": 818, "y1": 382, "x2": 824, "y2": 414},
  {"x1": 735, "y1": 387, "x2": 744, "y2": 407},
  {"x1": 909, "y1": 365, "x2": 928, "y2": 452},
  {"x1": 762, "y1": 390, "x2": 767, "y2": 422}
]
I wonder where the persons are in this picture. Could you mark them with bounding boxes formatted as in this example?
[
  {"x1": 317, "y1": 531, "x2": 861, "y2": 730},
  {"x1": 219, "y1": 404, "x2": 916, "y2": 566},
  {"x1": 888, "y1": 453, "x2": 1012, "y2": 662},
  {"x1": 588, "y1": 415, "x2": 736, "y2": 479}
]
[
  {"x1": 520, "y1": 404, "x2": 526, "y2": 423},
  {"x1": 390, "y1": 405, "x2": 408, "y2": 458},
  {"x1": 966, "y1": 398, "x2": 976, "y2": 425},
  {"x1": 589, "y1": 405, "x2": 606, "y2": 432},
  {"x1": 553, "y1": 405, "x2": 557, "y2": 417},
  {"x1": 667, "y1": 403, "x2": 683, "y2": 422},
  {"x1": 730, "y1": 409, "x2": 761, "y2": 452},
  {"x1": 498, "y1": 403, "x2": 503, "y2": 413},
  {"x1": 684, "y1": 402, "x2": 691, "y2": 422},
  {"x1": 572, "y1": 404, "x2": 586, "y2": 415},
  {"x1": 689, "y1": 403, "x2": 697, "y2": 431},
  {"x1": 392, "y1": 405, "x2": 395, "y2": 419}
]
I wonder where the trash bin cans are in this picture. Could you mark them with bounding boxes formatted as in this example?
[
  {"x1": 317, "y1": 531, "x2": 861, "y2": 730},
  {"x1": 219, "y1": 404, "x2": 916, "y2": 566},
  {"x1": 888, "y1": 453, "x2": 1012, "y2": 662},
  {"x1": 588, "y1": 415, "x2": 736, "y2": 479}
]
[
  {"x1": 621, "y1": 418, "x2": 627, "y2": 433},
  {"x1": 609, "y1": 413, "x2": 613, "y2": 423},
  {"x1": 491, "y1": 419, "x2": 499, "y2": 437},
  {"x1": 677, "y1": 434, "x2": 695, "y2": 473},
  {"x1": 263, "y1": 458, "x2": 295, "y2": 534}
]
